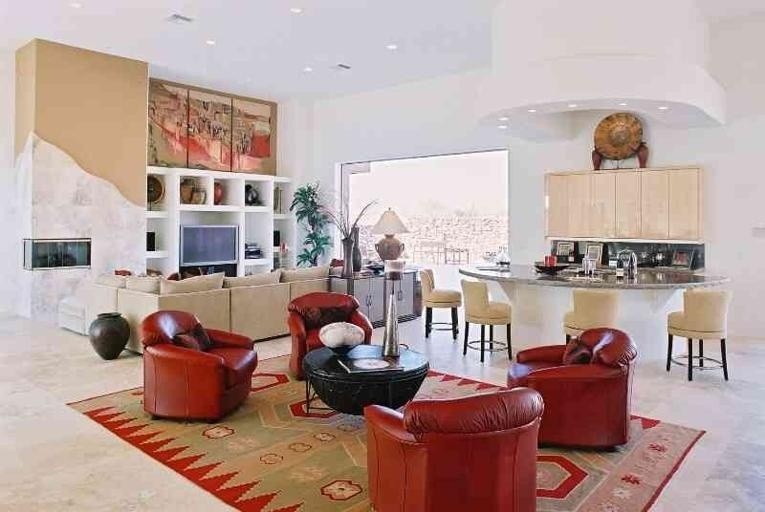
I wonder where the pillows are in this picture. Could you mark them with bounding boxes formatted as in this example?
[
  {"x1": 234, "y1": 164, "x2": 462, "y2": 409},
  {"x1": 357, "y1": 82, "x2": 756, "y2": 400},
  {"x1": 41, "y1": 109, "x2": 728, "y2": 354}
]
[
  {"x1": 560, "y1": 337, "x2": 592, "y2": 365},
  {"x1": 317, "y1": 319, "x2": 366, "y2": 348},
  {"x1": 171, "y1": 321, "x2": 212, "y2": 353},
  {"x1": 293, "y1": 305, "x2": 357, "y2": 326}
]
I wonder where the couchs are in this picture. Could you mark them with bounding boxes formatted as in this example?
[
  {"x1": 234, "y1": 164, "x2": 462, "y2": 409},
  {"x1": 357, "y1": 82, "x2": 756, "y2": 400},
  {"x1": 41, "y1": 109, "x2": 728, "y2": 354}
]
[{"x1": 56, "y1": 257, "x2": 425, "y2": 355}]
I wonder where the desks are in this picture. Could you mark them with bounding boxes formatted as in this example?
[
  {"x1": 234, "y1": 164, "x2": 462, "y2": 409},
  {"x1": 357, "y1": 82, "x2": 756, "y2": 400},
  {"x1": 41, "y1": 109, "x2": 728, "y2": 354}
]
[
  {"x1": 302, "y1": 342, "x2": 431, "y2": 414},
  {"x1": 457, "y1": 265, "x2": 731, "y2": 355}
]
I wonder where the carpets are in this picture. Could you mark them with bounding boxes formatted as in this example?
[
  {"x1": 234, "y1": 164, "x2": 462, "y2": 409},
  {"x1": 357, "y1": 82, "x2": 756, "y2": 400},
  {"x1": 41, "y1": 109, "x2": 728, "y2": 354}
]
[{"x1": 64, "y1": 343, "x2": 708, "y2": 511}]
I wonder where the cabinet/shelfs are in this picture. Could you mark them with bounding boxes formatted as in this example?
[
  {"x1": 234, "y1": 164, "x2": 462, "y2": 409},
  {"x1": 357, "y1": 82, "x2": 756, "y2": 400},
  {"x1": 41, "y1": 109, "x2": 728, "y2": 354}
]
[
  {"x1": 642, "y1": 167, "x2": 704, "y2": 242},
  {"x1": 591, "y1": 170, "x2": 642, "y2": 241},
  {"x1": 146, "y1": 165, "x2": 298, "y2": 276},
  {"x1": 543, "y1": 172, "x2": 590, "y2": 239}
]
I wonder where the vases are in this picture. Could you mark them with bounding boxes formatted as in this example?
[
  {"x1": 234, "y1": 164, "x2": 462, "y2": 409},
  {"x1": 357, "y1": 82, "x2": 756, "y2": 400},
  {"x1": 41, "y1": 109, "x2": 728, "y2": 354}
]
[
  {"x1": 341, "y1": 237, "x2": 354, "y2": 279},
  {"x1": 351, "y1": 226, "x2": 362, "y2": 272}
]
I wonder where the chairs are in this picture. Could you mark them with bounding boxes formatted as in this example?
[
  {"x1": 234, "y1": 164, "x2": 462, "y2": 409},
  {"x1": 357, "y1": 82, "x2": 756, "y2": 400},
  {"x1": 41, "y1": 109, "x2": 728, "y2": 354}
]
[
  {"x1": 418, "y1": 269, "x2": 463, "y2": 340},
  {"x1": 138, "y1": 308, "x2": 258, "y2": 421},
  {"x1": 665, "y1": 288, "x2": 735, "y2": 381},
  {"x1": 505, "y1": 326, "x2": 638, "y2": 451},
  {"x1": 561, "y1": 288, "x2": 624, "y2": 344},
  {"x1": 287, "y1": 289, "x2": 373, "y2": 381},
  {"x1": 460, "y1": 278, "x2": 514, "y2": 362},
  {"x1": 360, "y1": 385, "x2": 546, "y2": 510},
  {"x1": 443, "y1": 234, "x2": 471, "y2": 265}
]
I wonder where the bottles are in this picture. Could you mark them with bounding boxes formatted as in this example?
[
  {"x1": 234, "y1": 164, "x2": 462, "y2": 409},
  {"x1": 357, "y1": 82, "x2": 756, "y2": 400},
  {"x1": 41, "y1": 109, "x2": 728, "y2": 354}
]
[
  {"x1": 569, "y1": 246, "x2": 638, "y2": 279},
  {"x1": 214, "y1": 181, "x2": 223, "y2": 204},
  {"x1": 245, "y1": 184, "x2": 259, "y2": 206},
  {"x1": 496, "y1": 245, "x2": 511, "y2": 265},
  {"x1": 180, "y1": 176, "x2": 207, "y2": 205},
  {"x1": 88, "y1": 313, "x2": 130, "y2": 360}
]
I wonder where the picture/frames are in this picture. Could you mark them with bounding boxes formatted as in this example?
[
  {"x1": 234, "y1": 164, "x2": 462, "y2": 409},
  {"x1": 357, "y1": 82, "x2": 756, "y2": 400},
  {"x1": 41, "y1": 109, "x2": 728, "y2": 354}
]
[
  {"x1": 583, "y1": 241, "x2": 604, "y2": 268},
  {"x1": 145, "y1": 78, "x2": 280, "y2": 176},
  {"x1": 556, "y1": 242, "x2": 575, "y2": 256},
  {"x1": 671, "y1": 247, "x2": 698, "y2": 269}
]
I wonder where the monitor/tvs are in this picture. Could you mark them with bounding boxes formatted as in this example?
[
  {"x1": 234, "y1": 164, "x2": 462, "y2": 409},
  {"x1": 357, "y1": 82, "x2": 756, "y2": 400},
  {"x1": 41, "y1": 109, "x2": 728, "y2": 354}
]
[{"x1": 180, "y1": 225, "x2": 239, "y2": 267}]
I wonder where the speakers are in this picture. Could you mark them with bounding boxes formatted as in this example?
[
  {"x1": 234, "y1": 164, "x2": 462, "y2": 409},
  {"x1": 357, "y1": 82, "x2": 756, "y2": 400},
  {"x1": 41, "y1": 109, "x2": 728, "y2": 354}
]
[
  {"x1": 147, "y1": 232, "x2": 155, "y2": 251},
  {"x1": 274, "y1": 231, "x2": 280, "y2": 246}
]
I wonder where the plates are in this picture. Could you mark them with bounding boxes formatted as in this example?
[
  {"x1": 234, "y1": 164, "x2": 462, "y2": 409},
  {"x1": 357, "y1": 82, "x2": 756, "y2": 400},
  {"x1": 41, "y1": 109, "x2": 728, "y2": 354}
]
[
  {"x1": 594, "y1": 111, "x2": 644, "y2": 159},
  {"x1": 366, "y1": 265, "x2": 384, "y2": 270},
  {"x1": 146, "y1": 175, "x2": 164, "y2": 205},
  {"x1": 535, "y1": 265, "x2": 570, "y2": 273}
]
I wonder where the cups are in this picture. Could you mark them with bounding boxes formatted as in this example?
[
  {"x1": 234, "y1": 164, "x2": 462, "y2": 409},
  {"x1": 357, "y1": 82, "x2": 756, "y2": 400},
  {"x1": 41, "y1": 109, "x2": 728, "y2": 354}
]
[
  {"x1": 557, "y1": 254, "x2": 568, "y2": 263},
  {"x1": 544, "y1": 256, "x2": 556, "y2": 267}
]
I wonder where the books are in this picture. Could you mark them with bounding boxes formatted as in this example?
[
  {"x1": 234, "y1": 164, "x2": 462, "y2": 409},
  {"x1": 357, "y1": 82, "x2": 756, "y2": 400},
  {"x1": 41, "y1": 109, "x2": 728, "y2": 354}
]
[{"x1": 336, "y1": 356, "x2": 405, "y2": 374}]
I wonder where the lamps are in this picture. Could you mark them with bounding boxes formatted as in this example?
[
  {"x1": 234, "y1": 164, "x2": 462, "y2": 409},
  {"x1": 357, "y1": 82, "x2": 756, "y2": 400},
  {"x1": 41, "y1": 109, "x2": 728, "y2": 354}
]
[
  {"x1": 381, "y1": 258, "x2": 405, "y2": 357},
  {"x1": 369, "y1": 204, "x2": 412, "y2": 260}
]
